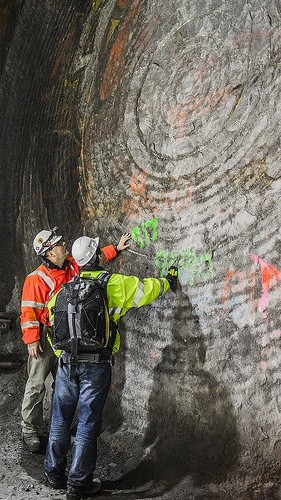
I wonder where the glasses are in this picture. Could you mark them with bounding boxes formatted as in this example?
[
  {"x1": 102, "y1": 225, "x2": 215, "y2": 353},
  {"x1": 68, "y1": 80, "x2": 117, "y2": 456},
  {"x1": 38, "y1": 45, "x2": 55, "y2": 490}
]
[{"x1": 44, "y1": 239, "x2": 63, "y2": 253}]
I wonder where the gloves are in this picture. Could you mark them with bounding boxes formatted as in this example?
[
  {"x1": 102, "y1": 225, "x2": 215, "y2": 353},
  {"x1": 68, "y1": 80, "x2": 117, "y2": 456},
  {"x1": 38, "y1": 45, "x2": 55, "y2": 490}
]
[{"x1": 165, "y1": 266, "x2": 177, "y2": 287}]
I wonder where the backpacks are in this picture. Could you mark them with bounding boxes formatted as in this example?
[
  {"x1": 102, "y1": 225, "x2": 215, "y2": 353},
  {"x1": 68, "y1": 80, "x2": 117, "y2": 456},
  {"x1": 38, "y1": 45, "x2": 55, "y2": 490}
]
[{"x1": 52, "y1": 272, "x2": 118, "y2": 353}]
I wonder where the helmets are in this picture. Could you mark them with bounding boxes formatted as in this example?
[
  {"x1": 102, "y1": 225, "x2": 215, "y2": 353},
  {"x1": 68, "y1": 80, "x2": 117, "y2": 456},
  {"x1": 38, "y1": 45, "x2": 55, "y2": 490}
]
[
  {"x1": 32, "y1": 230, "x2": 62, "y2": 256},
  {"x1": 72, "y1": 236, "x2": 100, "y2": 267}
]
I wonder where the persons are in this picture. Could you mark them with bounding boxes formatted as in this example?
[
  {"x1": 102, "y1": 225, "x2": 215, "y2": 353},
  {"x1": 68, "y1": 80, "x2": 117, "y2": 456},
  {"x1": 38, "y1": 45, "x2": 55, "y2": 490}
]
[
  {"x1": 43, "y1": 235, "x2": 178, "y2": 500},
  {"x1": 19, "y1": 226, "x2": 131, "y2": 451}
]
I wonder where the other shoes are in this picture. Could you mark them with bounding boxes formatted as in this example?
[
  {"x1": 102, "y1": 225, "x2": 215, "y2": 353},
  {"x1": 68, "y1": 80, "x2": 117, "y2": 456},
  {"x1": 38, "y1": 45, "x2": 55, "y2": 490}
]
[
  {"x1": 44, "y1": 472, "x2": 65, "y2": 487},
  {"x1": 67, "y1": 478, "x2": 101, "y2": 499},
  {"x1": 21, "y1": 431, "x2": 41, "y2": 451}
]
